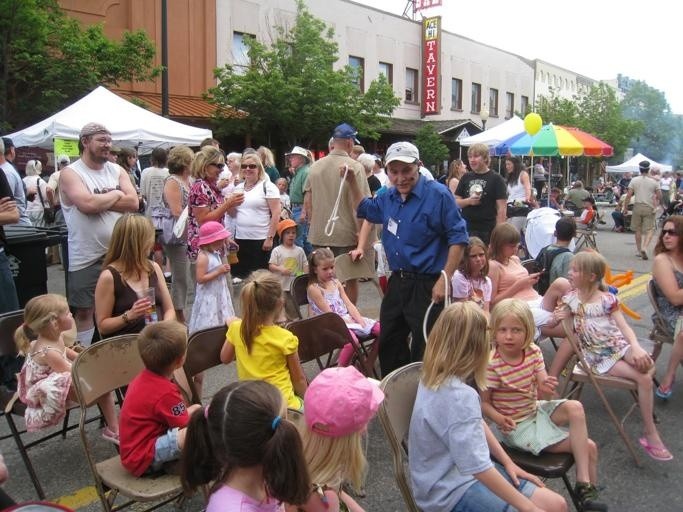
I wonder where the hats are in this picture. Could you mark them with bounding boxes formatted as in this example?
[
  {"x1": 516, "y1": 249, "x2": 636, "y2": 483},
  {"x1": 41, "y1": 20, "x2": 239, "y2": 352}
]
[
  {"x1": 285, "y1": 145, "x2": 312, "y2": 161},
  {"x1": 384, "y1": 141, "x2": 420, "y2": 166},
  {"x1": 305, "y1": 365, "x2": 385, "y2": 436},
  {"x1": 197, "y1": 221, "x2": 232, "y2": 249},
  {"x1": 277, "y1": 219, "x2": 298, "y2": 240},
  {"x1": 582, "y1": 195, "x2": 596, "y2": 204},
  {"x1": 333, "y1": 123, "x2": 358, "y2": 137}
]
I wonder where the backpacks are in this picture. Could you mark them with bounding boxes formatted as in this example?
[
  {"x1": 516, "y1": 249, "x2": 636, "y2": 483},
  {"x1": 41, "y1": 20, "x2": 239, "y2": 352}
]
[{"x1": 536, "y1": 244, "x2": 569, "y2": 297}]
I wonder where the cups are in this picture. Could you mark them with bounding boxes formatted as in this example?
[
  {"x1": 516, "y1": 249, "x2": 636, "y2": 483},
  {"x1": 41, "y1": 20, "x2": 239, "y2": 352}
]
[
  {"x1": 138, "y1": 288, "x2": 156, "y2": 314},
  {"x1": 231, "y1": 187, "x2": 244, "y2": 195}
]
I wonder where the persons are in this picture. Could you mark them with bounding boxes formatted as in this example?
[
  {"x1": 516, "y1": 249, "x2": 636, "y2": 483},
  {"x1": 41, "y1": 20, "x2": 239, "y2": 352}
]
[
  {"x1": 651, "y1": 213, "x2": 681, "y2": 334},
  {"x1": 604, "y1": 160, "x2": 681, "y2": 259},
  {"x1": 656, "y1": 316, "x2": 683, "y2": 397},
  {"x1": 407, "y1": 296, "x2": 568, "y2": 511},
  {"x1": 480, "y1": 297, "x2": 610, "y2": 512},
  {"x1": 545, "y1": 250, "x2": 673, "y2": 462},
  {"x1": 287, "y1": 365, "x2": 385, "y2": 512},
  {"x1": 1, "y1": 124, "x2": 314, "y2": 480},
  {"x1": 179, "y1": 377, "x2": 309, "y2": 512},
  {"x1": 505, "y1": 156, "x2": 606, "y2": 229},
  {"x1": 304, "y1": 125, "x2": 576, "y2": 373}
]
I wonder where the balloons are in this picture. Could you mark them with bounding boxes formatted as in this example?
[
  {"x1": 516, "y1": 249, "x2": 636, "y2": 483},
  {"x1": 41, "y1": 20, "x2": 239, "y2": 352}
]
[{"x1": 523, "y1": 112, "x2": 543, "y2": 137}]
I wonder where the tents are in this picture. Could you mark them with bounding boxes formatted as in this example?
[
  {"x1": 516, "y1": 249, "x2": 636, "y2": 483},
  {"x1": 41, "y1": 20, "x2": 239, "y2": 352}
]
[
  {"x1": 0, "y1": 85, "x2": 213, "y2": 176},
  {"x1": 605, "y1": 152, "x2": 672, "y2": 176}
]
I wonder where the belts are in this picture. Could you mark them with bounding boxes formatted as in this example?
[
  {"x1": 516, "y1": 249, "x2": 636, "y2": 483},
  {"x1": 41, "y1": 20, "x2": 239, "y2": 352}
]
[{"x1": 392, "y1": 270, "x2": 437, "y2": 282}]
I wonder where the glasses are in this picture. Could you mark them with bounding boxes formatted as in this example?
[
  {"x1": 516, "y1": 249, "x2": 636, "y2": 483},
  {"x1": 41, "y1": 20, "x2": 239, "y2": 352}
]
[
  {"x1": 241, "y1": 163, "x2": 257, "y2": 171},
  {"x1": 209, "y1": 162, "x2": 226, "y2": 168},
  {"x1": 662, "y1": 229, "x2": 678, "y2": 236}
]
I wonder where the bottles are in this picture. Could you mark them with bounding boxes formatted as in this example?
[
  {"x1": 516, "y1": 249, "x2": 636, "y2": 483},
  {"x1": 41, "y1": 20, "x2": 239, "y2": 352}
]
[{"x1": 227, "y1": 247, "x2": 238, "y2": 264}]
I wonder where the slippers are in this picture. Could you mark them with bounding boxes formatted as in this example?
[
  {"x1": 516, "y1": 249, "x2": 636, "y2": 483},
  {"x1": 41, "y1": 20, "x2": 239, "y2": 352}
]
[
  {"x1": 655, "y1": 386, "x2": 672, "y2": 399},
  {"x1": 639, "y1": 436, "x2": 674, "y2": 462}
]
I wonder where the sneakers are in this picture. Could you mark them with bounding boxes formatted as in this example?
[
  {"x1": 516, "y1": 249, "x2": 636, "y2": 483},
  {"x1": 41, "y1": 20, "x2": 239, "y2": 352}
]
[
  {"x1": 635, "y1": 249, "x2": 649, "y2": 260},
  {"x1": 101, "y1": 427, "x2": 121, "y2": 445},
  {"x1": 572, "y1": 481, "x2": 608, "y2": 512}
]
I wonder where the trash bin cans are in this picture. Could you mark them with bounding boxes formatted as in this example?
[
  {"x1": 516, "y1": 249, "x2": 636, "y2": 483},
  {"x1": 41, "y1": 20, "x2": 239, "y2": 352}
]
[
  {"x1": 2, "y1": 224, "x2": 68, "y2": 309},
  {"x1": 51, "y1": 224, "x2": 69, "y2": 303}
]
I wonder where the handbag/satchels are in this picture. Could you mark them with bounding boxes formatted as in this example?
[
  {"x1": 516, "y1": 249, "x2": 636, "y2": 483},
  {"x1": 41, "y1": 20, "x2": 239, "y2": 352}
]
[{"x1": 44, "y1": 208, "x2": 55, "y2": 222}]
